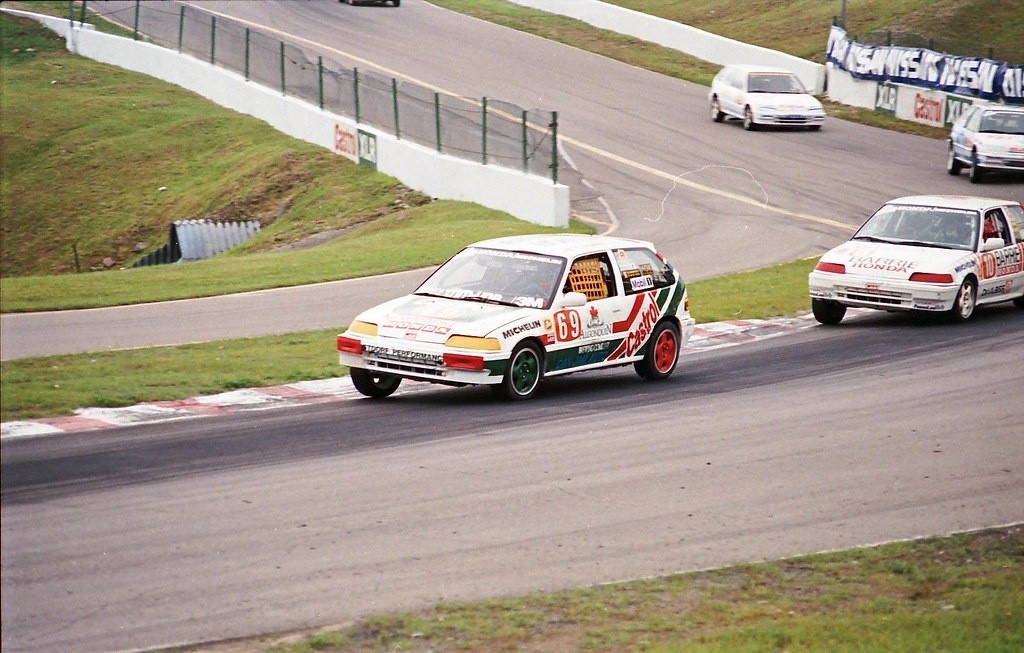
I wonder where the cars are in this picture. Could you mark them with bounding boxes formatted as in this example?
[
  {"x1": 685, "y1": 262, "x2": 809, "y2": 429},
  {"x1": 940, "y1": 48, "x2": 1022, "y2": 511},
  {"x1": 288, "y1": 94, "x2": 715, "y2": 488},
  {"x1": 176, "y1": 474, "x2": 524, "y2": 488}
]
[
  {"x1": 707, "y1": 64, "x2": 827, "y2": 132},
  {"x1": 808, "y1": 194, "x2": 1024, "y2": 325},
  {"x1": 335, "y1": 232, "x2": 697, "y2": 402},
  {"x1": 946, "y1": 103, "x2": 1024, "y2": 184}
]
